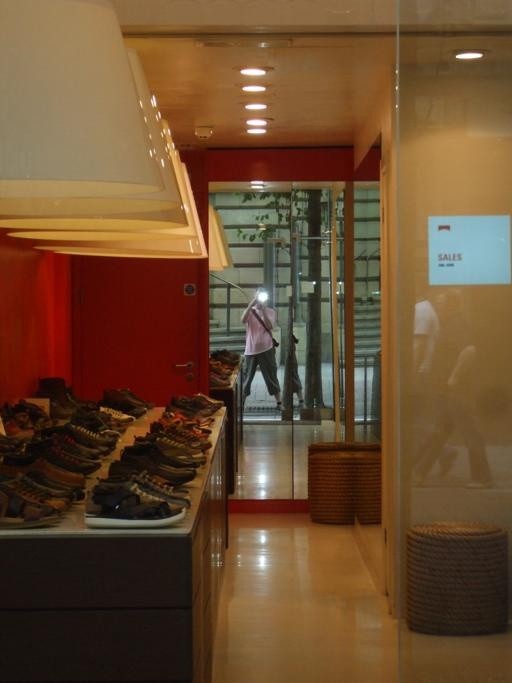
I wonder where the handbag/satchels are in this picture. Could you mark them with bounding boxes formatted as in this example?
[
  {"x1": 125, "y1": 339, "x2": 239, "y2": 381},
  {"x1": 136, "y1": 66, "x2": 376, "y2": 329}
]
[{"x1": 268, "y1": 331, "x2": 279, "y2": 348}]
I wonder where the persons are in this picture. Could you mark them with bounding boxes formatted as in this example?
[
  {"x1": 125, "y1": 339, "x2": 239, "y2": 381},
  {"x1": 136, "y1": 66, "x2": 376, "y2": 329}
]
[
  {"x1": 292, "y1": 335, "x2": 305, "y2": 411},
  {"x1": 403, "y1": 279, "x2": 461, "y2": 399},
  {"x1": 238, "y1": 287, "x2": 282, "y2": 411},
  {"x1": 411, "y1": 331, "x2": 498, "y2": 487}
]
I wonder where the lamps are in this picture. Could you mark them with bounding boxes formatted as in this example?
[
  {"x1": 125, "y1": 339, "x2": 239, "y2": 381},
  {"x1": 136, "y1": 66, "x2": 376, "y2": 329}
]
[
  {"x1": 29, "y1": 91, "x2": 194, "y2": 251},
  {"x1": 48, "y1": 161, "x2": 208, "y2": 259},
  {"x1": 0, "y1": 2, "x2": 167, "y2": 199},
  {"x1": 2, "y1": 46, "x2": 189, "y2": 232}
]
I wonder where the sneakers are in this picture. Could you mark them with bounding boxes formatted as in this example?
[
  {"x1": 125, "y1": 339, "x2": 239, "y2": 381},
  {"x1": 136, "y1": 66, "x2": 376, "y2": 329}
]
[
  {"x1": 0, "y1": 376, "x2": 149, "y2": 529},
  {"x1": 277, "y1": 402, "x2": 283, "y2": 412},
  {"x1": 208, "y1": 348, "x2": 240, "y2": 387},
  {"x1": 84, "y1": 390, "x2": 224, "y2": 528}
]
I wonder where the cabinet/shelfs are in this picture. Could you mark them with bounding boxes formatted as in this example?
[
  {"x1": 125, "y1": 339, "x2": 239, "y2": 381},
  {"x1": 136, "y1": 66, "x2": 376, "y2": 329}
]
[{"x1": 0, "y1": 399, "x2": 230, "y2": 683}]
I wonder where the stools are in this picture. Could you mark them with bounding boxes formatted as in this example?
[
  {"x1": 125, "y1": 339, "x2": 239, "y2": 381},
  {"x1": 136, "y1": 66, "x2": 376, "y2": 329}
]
[
  {"x1": 403, "y1": 517, "x2": 512, "y2": 639},
  {"x1": 303, "y1": 440, "x2": 384, "y2": 528}
]
